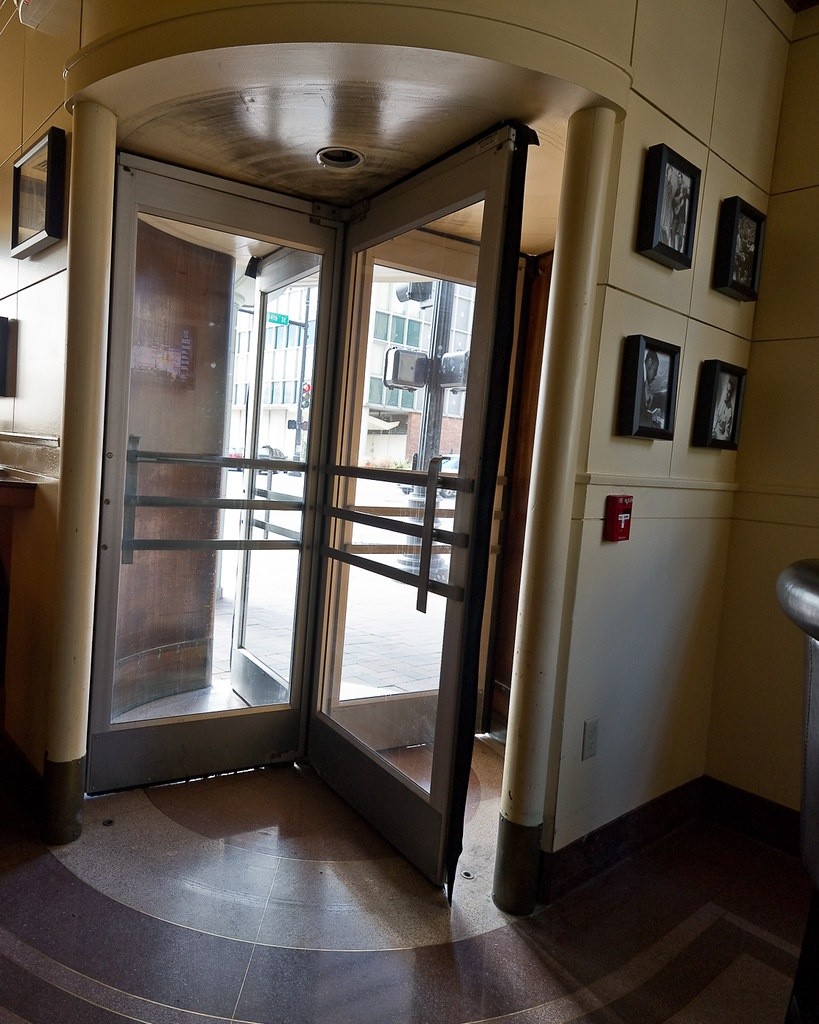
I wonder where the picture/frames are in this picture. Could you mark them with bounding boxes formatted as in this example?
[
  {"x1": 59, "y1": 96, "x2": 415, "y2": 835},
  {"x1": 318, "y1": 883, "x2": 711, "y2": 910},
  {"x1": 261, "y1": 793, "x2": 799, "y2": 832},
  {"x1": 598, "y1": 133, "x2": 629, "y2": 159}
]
[
  {"x1": 614, "y1": 333, "x2": 682, "y2": 442},
  {"x1": 634, "y1": 143, "x2": 703, "y2": 271},
  {"x1": 710, "y1": 196, "x2": 767, "y2": 303},
  {"x1": 10, "y1": 126, "x2": 65, "y2": 260},
  {"x1": 689, "y1": 358, "x2": 749, "y2": 451}
]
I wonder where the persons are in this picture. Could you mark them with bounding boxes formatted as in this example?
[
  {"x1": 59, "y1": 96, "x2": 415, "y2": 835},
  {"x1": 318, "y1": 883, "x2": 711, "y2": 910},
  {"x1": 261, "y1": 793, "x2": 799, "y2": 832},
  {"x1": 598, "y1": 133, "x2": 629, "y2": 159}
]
[
  {"x1": 642, "y1": 349, "x2": 669, "y2": 429},
  {"x1": 713, "y1": 376, "x2": 739, "y2": 445},
  {"x1": 736, "y1": 226, "x2": 757, "y2": 285},
  {"x1": 661, "y1": 162, "x2": 690, "y2": 251}
]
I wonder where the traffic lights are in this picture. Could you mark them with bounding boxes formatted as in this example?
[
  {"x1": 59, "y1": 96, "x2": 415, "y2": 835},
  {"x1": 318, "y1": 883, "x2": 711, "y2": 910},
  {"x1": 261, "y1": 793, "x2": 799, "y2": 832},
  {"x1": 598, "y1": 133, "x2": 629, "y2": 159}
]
[
  {"x1": 396, "y1": 281, "x2": 430, "y2": 303},
  {"x1": 301, "y1": 381, "x2": 311, "y2": 409}
]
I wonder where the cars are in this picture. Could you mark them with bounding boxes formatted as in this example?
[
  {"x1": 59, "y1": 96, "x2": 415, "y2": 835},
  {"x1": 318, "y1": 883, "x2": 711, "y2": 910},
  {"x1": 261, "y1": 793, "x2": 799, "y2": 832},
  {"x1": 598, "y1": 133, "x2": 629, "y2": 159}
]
[
  {"x1": 399, "y1": 453, "x2": 460, "y2": 498},
  {"x1": 258, "y1": 447, "x2": 288, "y2": 475},
  {"x1": 228, "y1": 448, "x2": 241, "y2": 470}
]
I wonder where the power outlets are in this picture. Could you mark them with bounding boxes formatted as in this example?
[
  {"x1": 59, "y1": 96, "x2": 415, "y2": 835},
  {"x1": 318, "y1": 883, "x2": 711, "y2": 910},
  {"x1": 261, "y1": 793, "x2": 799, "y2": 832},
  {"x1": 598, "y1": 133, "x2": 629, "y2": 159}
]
[{"x1": 582, "y1": 716, "x2": 600, "y2": 760}]
